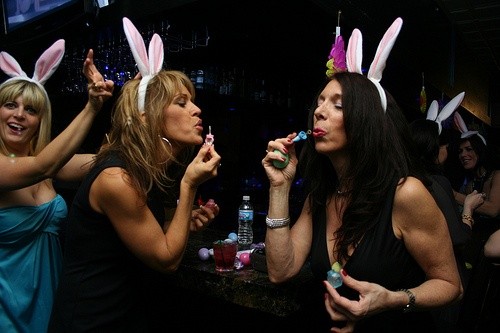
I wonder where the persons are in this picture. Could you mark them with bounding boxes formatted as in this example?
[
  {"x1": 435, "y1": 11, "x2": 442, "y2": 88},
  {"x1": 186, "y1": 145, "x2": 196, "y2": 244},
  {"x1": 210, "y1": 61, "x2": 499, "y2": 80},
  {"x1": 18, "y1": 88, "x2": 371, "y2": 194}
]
[
  {"x1": 50, "y1": 18, "x2": 223, "y2": 333},
  {"x1": 0, "y1": 40, "x2": 115, "y2": 333},
  {"x1": 394, "y1": 84, "x2": 500, "y2": 333},
  {"x1": 260, "y1": 17, "x2": 465, "y2": 333}
]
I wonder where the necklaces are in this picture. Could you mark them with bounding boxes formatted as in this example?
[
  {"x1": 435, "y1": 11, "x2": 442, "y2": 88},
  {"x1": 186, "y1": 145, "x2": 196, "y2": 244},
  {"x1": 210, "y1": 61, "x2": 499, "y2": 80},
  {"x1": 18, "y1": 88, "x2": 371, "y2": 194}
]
[{"x1": 334, "y1": 179, "x2": 354, "y2": 197}]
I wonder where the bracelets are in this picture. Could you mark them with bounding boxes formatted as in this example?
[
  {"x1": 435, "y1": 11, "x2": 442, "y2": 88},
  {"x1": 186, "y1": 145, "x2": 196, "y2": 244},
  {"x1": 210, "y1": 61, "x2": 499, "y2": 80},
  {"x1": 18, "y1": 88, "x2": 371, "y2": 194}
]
[
  {"x1": 461, "y1": 214, "x2": 476, "y2": 227},
  {"x1": 265, "y1": 214, "x2": 291, "y2": 228}
]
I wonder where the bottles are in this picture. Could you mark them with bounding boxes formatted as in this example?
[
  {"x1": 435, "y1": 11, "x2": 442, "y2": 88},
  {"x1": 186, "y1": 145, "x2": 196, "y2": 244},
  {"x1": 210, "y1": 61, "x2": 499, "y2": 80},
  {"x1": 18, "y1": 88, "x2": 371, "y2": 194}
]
[
  {"x1": 238, "y1": 196, "x2": 253, "y2": 244},
  {"x1": 186, "y1": 63, "x2": 293, "y2": 108}
]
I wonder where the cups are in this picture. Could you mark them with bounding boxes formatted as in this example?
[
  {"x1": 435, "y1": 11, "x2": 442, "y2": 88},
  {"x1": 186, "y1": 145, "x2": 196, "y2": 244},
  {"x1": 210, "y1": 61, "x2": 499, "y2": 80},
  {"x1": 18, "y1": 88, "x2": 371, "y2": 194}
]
[{"x1": 212, "y1": 242, "x2": 237, "y2": 272}]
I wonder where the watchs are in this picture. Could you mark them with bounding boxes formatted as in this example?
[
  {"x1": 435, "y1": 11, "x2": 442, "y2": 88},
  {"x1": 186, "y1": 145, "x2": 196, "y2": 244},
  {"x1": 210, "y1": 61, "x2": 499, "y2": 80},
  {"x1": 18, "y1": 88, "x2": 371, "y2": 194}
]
[{"x1": 396, "y1": 288, "x2": 416, "y2": 314}]
